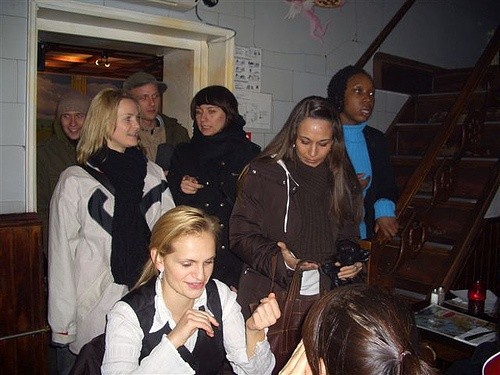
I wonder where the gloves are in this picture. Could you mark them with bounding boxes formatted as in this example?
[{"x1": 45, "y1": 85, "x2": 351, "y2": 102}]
[{"x1": 323, "y1": 242, "x2": 370, "y2": 290}]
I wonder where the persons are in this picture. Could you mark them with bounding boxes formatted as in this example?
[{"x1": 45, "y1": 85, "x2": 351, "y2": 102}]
[
  {"x1": 228, "y1": 95, "x2": 368, "y2": 375},
  {"x1": 165, "y1": 85, "x2": 262, "y2": 272},
  {"x1": 36, "y1": 88, "x2": 86, "y2": 215},
  {"x1": 47, "y1": 87, "x2": 176, "y2": 375},
  {"x1": 123, "y1": 72, "x2": 191, "y2": 164},
  {"x1": 100, "y1": 205, "x2": 281, "y2": 375},
  {"x1": 326, "y1": 65, "x2": 399, "y2": 242},
  {"x1": 277, "y1": 284, "x2": 436, "y2": 375}
]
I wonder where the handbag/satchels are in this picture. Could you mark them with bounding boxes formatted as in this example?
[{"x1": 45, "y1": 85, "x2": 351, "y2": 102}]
[{"x1": 249, "y1": 259, "x2": 329, "y2": 375}]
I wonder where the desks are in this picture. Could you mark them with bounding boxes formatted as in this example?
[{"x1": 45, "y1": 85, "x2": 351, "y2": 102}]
[{"x1": 410, "y1": 297, "x2": 500, "y2": 375}]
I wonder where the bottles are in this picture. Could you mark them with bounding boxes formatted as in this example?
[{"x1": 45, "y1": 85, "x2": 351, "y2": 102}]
[
  {"x1": 430, "y1": 287, "x2": 445, "y2": 306},
  {"x1": 467, "y1": 280, "x2": 485, "y2": 314}
]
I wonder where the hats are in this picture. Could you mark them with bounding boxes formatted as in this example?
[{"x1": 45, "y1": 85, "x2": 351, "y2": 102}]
[
  {"x1": 56, "y1": 92, "x2": 89, "y2": 124},
  {"x1": 123, "y1": 72, "x2": 167, "y2": 94}
]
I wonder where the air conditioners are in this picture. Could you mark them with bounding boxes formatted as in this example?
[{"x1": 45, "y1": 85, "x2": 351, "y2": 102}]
[{"x1": 116, "y1": 0, "x2": 201, "y2": 12}]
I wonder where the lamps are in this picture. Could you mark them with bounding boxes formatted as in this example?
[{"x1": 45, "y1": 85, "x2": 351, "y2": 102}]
[{"x1": 95, "y1": 54, "x2": 111, "y2": 67}]
